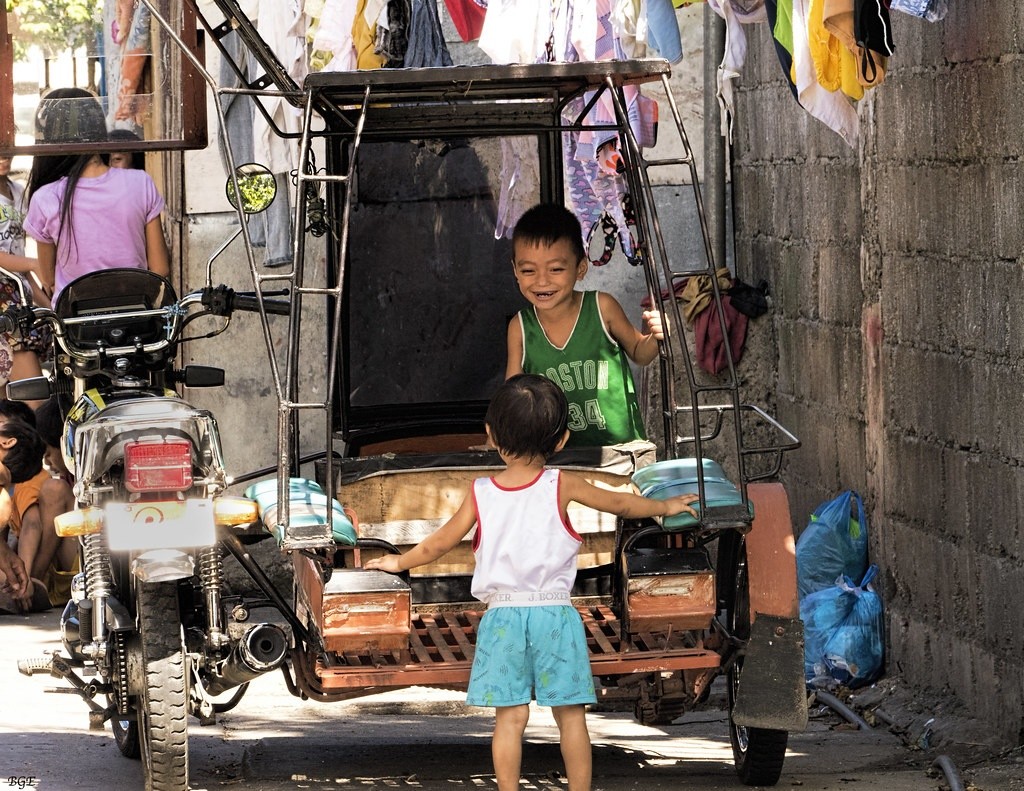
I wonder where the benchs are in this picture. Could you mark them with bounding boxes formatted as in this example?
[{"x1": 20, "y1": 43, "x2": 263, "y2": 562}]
[
  {"x1": 244, "y1": 477, "x2": 412, "y2": 654},
  {"x1": 612, "y1": 456, "x2": 755, "y2": 649}
]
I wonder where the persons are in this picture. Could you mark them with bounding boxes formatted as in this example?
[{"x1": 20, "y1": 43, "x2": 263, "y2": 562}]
[
  {"x1": 1, "y1": 396, "x2": 55, "y2": 611},
  {"x1": 15, "y1": 88, "x2": 173, "y2": 314},
  {"x1": 19, "y1": 393, "x2": 80, "y2": 618},
  {"x1": 1, "y1": 418, "x2": 46, "y2": 600},
  {"x1": 103, "y1": 128, "x2": 146, "y2": 171},
  {"x1": 358, "y1": 373, "x2": 703, "y2": 791},
  {"x1": 0, "y1": 157, "x2": 60, "y2": 410},
  {"x1": 465, "y1": 200, "x2": 672, "y2": 453}
]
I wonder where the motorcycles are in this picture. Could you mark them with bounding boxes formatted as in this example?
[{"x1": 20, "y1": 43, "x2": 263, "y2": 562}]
[{"x1": 0, "y1": 57, "x2": 806, "y2": 791}]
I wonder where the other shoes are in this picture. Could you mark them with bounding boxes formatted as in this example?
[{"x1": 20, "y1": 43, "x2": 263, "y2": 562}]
[
  {"x1": 30, "y1": 579, "x2": 53, "y2": 610},
  {"x1": 0, "y1": 570, "x2": 22, "y2": 613}
]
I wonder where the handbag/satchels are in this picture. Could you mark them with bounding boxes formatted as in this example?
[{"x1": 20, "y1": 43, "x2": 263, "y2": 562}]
[
  {"x1": 796, "y1": 491, "x2": 865, "y2": 600},
  {"x1": 799, "y1": 565, "x2": 882, "y2": 692}
]
